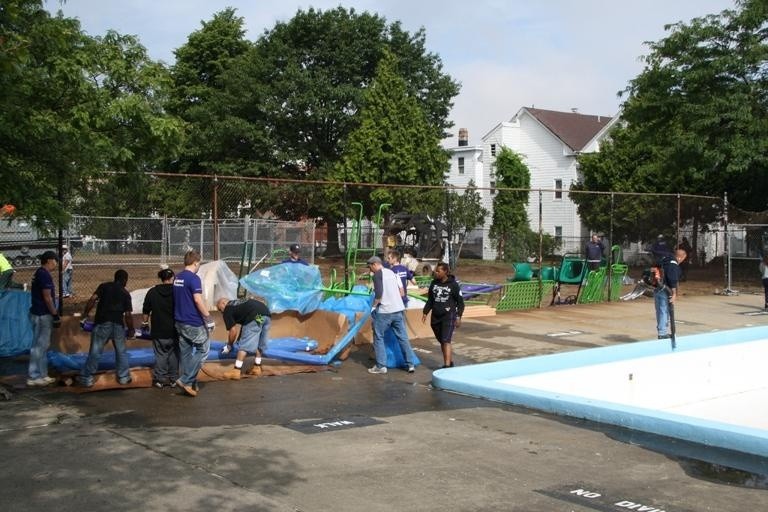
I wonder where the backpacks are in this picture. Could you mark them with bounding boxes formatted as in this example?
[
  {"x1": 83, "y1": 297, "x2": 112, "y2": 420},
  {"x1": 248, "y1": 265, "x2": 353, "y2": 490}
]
[{"x1": 639, "y1": 266, "x2": 665, "y2": 298}]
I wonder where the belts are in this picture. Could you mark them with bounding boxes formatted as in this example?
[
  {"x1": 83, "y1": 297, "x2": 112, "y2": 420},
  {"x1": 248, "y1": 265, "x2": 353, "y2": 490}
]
[{"x1": 32, "y1": 312, "x2": 51, "y2": 316}]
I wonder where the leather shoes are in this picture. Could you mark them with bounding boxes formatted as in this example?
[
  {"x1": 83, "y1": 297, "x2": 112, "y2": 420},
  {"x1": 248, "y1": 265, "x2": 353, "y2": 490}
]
[{"x1": 658, "y1": 334, "x2": 671, "y2": 339}]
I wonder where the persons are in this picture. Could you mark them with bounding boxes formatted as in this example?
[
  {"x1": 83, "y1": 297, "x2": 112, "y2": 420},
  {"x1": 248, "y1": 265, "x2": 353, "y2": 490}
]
[
  {"x1": 758, "y1": 254, "x2": 768, "y2": 312},
  {"x1": 217, "y1": 295, "x2": 272, "y2": 381},
  {"x1": 367, "y1": 256, "x2": 415, "y2": 374},
  {"x1": 172, "y1": 251, "x2": 216, "y2": 397},
  {"x1": 421, "y1": 261, "x2": 466, "y2": 368},
  {"x1": 0, "y1": 249, "x2": 28, "y2": 291},
  {"x1": 60, "y1": 245, "x2": 74, "y2": 298},
  {"x1": 26, "y1": 250, "x2": 58, "y2": 387},
  {"x1": 142, "y1": 269, "x2": 179, "y2": 389},
  {"x1": 585, "y1": 234, "x2": 602, "y2": 272},
  {"x1": 386, "y1": 252, "x2": 417, "y2": 328},
  {"x1": 278, "y1": 243, "x2": 309, "y2": 266},
  {"x1": 75, "y1": 268, "x2": 136, "y2": 388},
  {"x1": 654, "y1": 248, "x2": 688, "y2": 340}
]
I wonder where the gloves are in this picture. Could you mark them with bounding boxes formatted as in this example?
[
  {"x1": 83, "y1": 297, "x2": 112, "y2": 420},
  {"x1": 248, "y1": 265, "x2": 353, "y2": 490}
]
[
  {"x1": 233, "y1": 341, "x2": 239, "y2": 348},
  {"x1": 140, "y1": 321, "x2": 149, "y2": 337},
  {"x1": 205, "y1": 315, "x2": 215, "y2": 332},
  {"x1": 80, "y1": 313, "x2": 89, "y2": 328},
  {"x1": 370, "y1": 305, "x2": 377, "y2": 319},
  {"x1": 127, "y1": 328, "x2": 135, "y2": 338},
  {"x1": 53, "y1": 314, "x2": 61, "y2": 328},
  {"x1": 221, "y1": 344, "x2": 233, "y2": 355}
]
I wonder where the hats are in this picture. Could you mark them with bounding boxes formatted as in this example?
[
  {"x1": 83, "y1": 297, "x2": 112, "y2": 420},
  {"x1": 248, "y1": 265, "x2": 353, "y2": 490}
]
[
  {"x1": 367, "y1": 256, "x2": 382, "y2": 263},
  {"x1": 62, "y1": 245, "x2": 68, "y2": 248},
  {"x1": 43, "y1": 251, "x2": 59, "y2": 263},
  {"x1": 290, "y1": 244, "x2": 301, "y2": 252}
]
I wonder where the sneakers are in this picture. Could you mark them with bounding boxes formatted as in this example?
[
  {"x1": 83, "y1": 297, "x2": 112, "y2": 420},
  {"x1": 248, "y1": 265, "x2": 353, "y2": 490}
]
[
  {"x1": 368, "y1": 365, "x2": 388, "y2": 374},
  {"x1": 765, "y1": 304, "x2": 768, "y2": 308},
  {"x1": 153, "y1": 380, "x2": 163, "y2": 389},
  {"x1": 63, "y1": 294, "x2": 70, "y2": 298},
  {"x1": 169, "y1": 382, "x2": 176, "y2": 388},
  {"x1": 24, "y1": 283, "x2": 28, "y2": 291},
  {"x1": 176, "y1": 379, "x2": 197, "y2": 396},
  {"x1": 184, "y1": 385, "x2": 199, "y2": 395},
  {"x1": 128, "y1": 378, "x2": 132, "y2": 383},
  {"x1": 27, "y1": 377, "x2": 49, "y2": 386},
  {"x1": 223, "y1": 368, "x2": 241, "y2": 380},
  {"x1": 408, "y1": 368, "x2": 415, "y2": 373},
  {"x1": 442, "y1": 365, "x2": 450, "y2": 368},
  {"x1": 246, "y1": 364, "x2": 262, "y2": 376},
  {"x1": 43, "y1": 376, "x2": 56, "y2": 383},
  {"x1": 450, "y1": 361, "x2": 454, "y2": 367}
]
